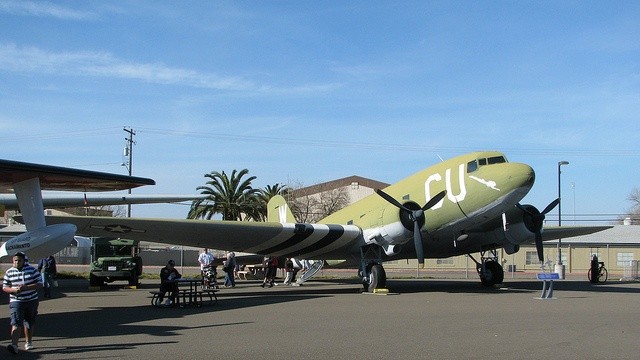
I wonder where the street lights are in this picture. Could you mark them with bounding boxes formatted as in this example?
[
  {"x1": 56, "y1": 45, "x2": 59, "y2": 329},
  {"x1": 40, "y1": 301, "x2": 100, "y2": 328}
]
[
  {"x1": 120, "y1": 163, "x2": 132, "y2": 218},
  {"x1": 556, "y1": 161, "x2": 570, "y2": 263}
]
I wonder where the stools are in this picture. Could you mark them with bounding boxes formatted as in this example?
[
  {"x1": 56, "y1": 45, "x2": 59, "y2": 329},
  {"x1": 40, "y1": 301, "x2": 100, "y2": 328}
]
[
  {"x1": 166, "y1": 289, "x2": 194, "y2": 305},
  {"x1": 203, "y1": 289, "x2": 220, "y2": 307},
  {"x1": 174, "y1": 292, "x2": 203, "y2": 307},
  {"x1": 150, "y1": 291, "x2": 163, "y2": 307}
]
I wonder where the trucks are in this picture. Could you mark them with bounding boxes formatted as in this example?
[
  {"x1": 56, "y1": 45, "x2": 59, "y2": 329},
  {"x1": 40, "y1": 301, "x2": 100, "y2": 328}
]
[{"x1": 90, "y1": 236, "x2": 142, "y2": 288}]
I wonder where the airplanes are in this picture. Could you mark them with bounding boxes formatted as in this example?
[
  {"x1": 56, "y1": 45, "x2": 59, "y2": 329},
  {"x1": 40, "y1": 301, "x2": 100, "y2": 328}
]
[
  {"x1": 11, "y1": 151, "x2": 614, "y2": 293},
  {"x1": 0, "y1": 158, "x2": 211, "y2": 263}
]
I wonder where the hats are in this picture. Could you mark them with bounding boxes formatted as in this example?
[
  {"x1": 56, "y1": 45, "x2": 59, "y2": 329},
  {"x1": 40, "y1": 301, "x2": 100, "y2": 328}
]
[{"x1": 167, "y1": 260, "x2": 176, "y2": 266}]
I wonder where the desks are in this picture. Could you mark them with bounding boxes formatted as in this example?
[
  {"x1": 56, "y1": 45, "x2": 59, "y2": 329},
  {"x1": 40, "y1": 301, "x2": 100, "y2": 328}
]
[{"x1": 164, "y1": 280, "x2": 204, "y2": 308}]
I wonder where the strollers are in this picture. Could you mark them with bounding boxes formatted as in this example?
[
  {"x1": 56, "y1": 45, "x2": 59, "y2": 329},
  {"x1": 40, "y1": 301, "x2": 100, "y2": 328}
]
[{"x1": 203, "y1": 265, "x2": 220, "y2": 290}]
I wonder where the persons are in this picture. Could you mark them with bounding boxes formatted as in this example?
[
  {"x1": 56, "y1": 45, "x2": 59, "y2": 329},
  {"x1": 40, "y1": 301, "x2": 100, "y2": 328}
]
[
  {"x1": 260, "y1": 257, "x2": 278, "y2": 288},
  {"x1": 222, "y1": 251, "x2": 236, "y2": 287},
  {"x1": 3, "y1": 253, "x2": 43, "y2": 354},
  {"x1": 284, "y1": 258, "x2": 294, "y2": 285},
  {"x1": 38, "y1": 256, "x2": 56, "y2": 300},
  {"x1": 157, "y1": 260, "x2": 181, "y2": 305},
  {"x1": 198, "y1": 248, "x2": 214, "y2": 289}
]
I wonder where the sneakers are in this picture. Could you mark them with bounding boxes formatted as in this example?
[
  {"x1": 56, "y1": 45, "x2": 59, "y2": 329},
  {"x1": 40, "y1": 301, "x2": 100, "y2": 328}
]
[
  {"x1": 24, "y1": 342, "x2": 34, "y2": 350},
  {"x1": 165, "y1": 299, "x2": 170, "y2": 305},
  {"x1": 157, "y1": 301, "x2": 161, "y2": 305},
  {"x1": 8, "y1": 344, "x2": 19, "y2": 353}
]
